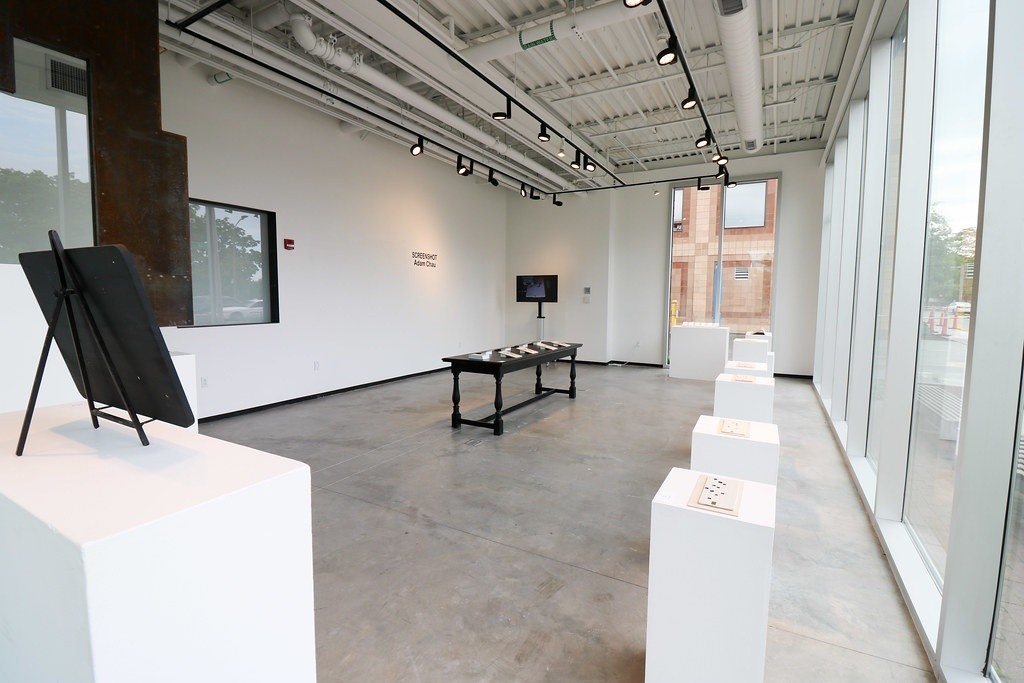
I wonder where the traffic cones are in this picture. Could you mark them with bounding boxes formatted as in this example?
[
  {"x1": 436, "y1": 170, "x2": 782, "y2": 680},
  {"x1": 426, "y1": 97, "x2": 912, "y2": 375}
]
[
  {"x1": 936, "y1": 313, "x2": 944, "y2": 326},
  {"x1": 949, "y1": 315, "x2": 959, "y2": 330},
  {"x1": 938, "y1": 318, "x2": 950, "y2": 336},
  {"x1": 927, "y1": 312, "x2": 935, "y2": 324},
  {"x1": 928, "y1": 319, "x2": 939, "y2": 334}
]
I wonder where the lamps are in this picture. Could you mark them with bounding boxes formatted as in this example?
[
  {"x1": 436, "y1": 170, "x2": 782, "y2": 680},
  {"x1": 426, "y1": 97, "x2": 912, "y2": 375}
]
[
  {"x1": 531, "y1": 188, "x2": 540, "y2": 200},
  {"x1": 461, "y1": 160, "x2": 475, "y2": 176},
  {"x1": 712, "y1": 153, "x2": 729, "y2": 166},
  {"x1": 723, "y1": 175, "x2": 738, "y2": 189},
  {"x1": 582, "y1": 156, "x2": 596, "y2": 172},
  {"x1": 715, "y1": 165, "x2": 726, "y2": 180},
  {"x1": 695, "y1": 129, "x2": 712, "y2": 149},
  {"x1": 622, "y1": 0, "x2": 652, "y2": 9},
  {"x1": 488, "y1": 170, "x2": 500, "y2": 186},
  {"x1": 553, "y1": 194, "x2": 563, "y2": 206},
  {"x1": 492, "y1": 98, "x2": 511, "y2": 120},
  {"x1": 410, "y1": 138, "x2": 424, "y2": 156},
  {"x1": 697, "y1": 178, "x2": 710, "y2": 190},
  {"x1": 520, "y1": 183, "x2": 527, "y2": 198},
  {"x1": 457, "y1": 155, "x2": 466, "y2": 174},
  {"x1": 537, "y1": 124, "x2": 550, "y2": 142},
  {"x1": 681, "y1": 88, "x2": 697, "y2": 110},
  {"x1": 656, "y1": 40, "x2": 680, "y2": 66},
  {"x1": 570, "y1": 149, "x2": 581, "y2": 170},
  {"x1": 557, "y1": 139, "x2": 565, "y2": 157}
]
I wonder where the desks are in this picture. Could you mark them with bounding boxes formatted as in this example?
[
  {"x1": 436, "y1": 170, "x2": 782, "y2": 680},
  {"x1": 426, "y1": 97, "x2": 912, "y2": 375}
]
[{"x1": 441, "y1": 340, "x2": 584, "y2": 436}]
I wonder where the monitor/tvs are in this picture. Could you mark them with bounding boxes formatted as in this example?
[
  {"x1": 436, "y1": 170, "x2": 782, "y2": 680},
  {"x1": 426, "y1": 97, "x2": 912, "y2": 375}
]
[{"x1": 516, "y1": 275, "x2": 558, "y2": 303}]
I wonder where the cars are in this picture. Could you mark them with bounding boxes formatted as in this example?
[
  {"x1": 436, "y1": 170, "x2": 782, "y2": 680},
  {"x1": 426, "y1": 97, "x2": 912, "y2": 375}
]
[{"x1": 947, "y1": 302, "x2": 971, "y2": 314}]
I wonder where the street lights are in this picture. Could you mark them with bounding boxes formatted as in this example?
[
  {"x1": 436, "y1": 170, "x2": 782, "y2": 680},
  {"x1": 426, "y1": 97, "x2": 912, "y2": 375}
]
[{"x1": 231, "y1": 215, "x2": 249, "y2": 307}]
